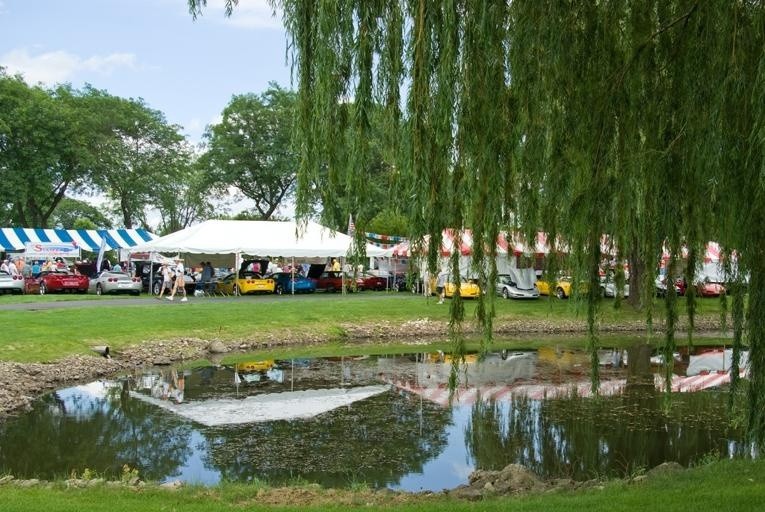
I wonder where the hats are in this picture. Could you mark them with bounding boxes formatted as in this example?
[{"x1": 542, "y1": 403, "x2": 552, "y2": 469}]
[
  {"x1": 160, "y1": 259, "x2": 169, "y2": 265},
  {"x1": 2, "y1": 255, "x2": 61, "y2": 265},
  {"x1": 172, "y1": 257, "x2": 181, "y2": 261}
]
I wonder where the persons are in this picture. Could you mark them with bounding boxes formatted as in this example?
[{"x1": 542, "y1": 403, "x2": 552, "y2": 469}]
[
  {"x1": 330, "y1": 258, "x2": 365, "y2": 278},
  {"x1": 105, "y1": 258, "x2": 138, "y2": 278},
  {"x1": 0, "y1": 255, "x2": 70, "y2": 277},
  {"x1": 150, "y1": 369, "x2": 186, "y2": 405},
  {"x1": 151, "y1": 255, "x2": 217, "y2": 302},
  {"x1": 422, "y1": 263, "x2": 449, "y2": 305},
  {"x1": 240, "y1": 255, "x2": 307, "y2": 278}
]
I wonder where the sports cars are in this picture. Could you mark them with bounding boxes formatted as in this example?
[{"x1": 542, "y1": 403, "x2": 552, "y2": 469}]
[
  {"x1": 488, "y1": 275, "x2": 540, "y2": 300},
  {"x1": 661, "y1": 272, "x2": 698, "y2": 297},
  {"x1": 434, "y1": 273, "x2": 481, "y2": 300},
  {"x1": 209, "y1": 259, "x2": 275, "y2": 295},
  {"x1": 0, "y1": 262, "x2": 25, "y2": 294},
  {"x1": 534, "y1": 275, "x2": 590, "y2": 299},
  {"x1": 88, "y1": 271, "x2": 144, "y2": 295},
  {"x1": 696, "y1": 281, "x2": 726, "y2": 296},
  {"x1": 653, "y1": 277, "x2": 683, "y2": 296},
  {"x1": 265, "y1": 268, "x2": 423, "y2": 294},
  {"x1": 23, "y1": 270, "x2": 89, "y2": 295},
  {"x1": 222, "y1": 347, "x2": 737, "y2": 385},
  {"x1": 140, "y1": 266, "x2": 197, "y2": 297},
  {"x1": 588, "y1": 276, "x2": 634, "y2": 297}
]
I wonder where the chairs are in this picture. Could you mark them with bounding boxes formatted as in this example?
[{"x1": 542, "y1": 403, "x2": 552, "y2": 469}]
[{"x1": 189, "y1": 277, "x2": 233, "y2": 299}]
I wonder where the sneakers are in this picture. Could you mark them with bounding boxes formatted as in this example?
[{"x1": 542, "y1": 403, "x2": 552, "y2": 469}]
[
  {"x1": 181, "y1": 297, "x2": 188, "y2": 301},
  {"x1": 155, "y1": 295, "x2": 162, "y2": 300},
  {"x1": 436, "y1": 292, "x2": 444, "y2": 306},
  {"x1": 165, "y1": 295, "x2": 174, "y2": 301}
]
